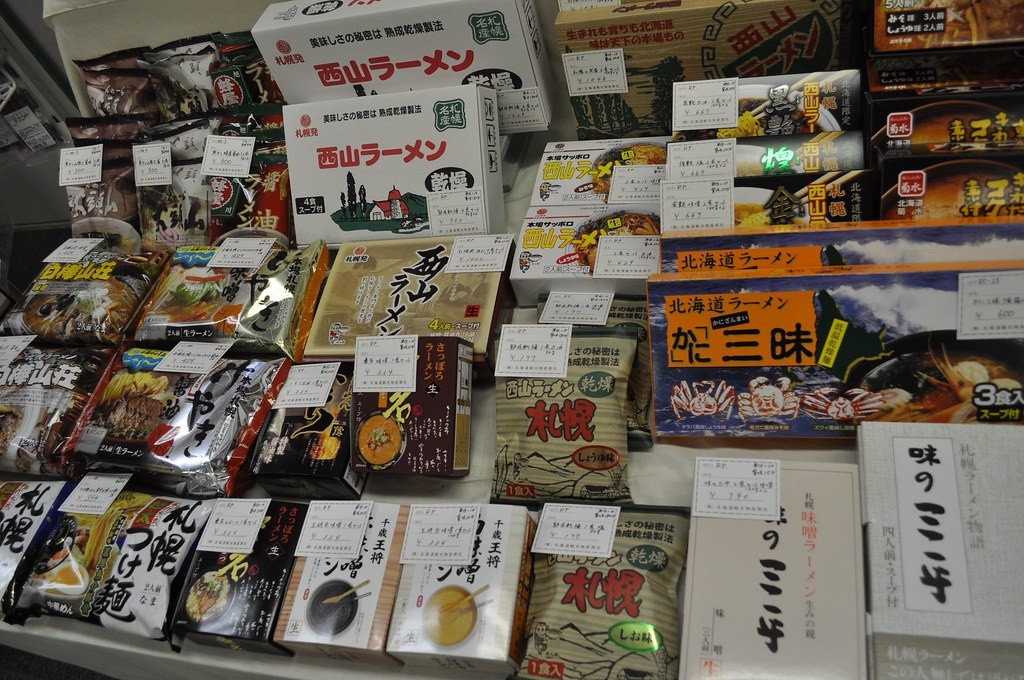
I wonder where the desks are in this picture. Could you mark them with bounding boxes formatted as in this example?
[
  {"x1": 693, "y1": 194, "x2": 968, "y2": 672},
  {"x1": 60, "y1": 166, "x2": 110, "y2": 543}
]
[{"x1": 0, "y1": 612, "x2": 440, "y2": 680}]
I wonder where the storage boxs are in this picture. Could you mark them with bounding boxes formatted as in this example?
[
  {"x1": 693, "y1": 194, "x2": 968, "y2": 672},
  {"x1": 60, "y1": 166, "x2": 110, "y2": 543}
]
[
  {"x1": 860, "y1": 0, "x2": 1024, "y2": 221},
  {"x1": 280, "y1": 83, "x2": 506, "y2": 251},
  {"x1": 556, "y1": 0, "x2": 841, "y2": 140},
  {"x1": 675, "y1": 457, "x2": 869, "y2": 680},
  {"x1": 251, "y1": 0, "x2": 554, "y2": 134},
  {"x1": 310, "y1": 68, "x2": 1024, "y2": 447},
  {"x1": 170, "y1": 494, "x2": 536, "y2": 674}
]
